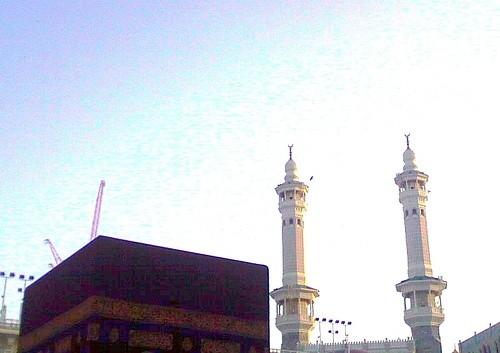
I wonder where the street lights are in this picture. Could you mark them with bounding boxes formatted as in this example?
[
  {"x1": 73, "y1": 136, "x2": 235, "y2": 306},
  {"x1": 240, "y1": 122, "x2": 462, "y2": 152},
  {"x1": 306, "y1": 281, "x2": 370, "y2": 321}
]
[
  {"x1": 328, "y1": 319, "x2": 340, "y2": 353},
  {"x1": 0, "y1": 272, "x2": 15, "y2": 327},
  {"x1": 17, "y1": 274, "x2": 34, "y2": 293},
  {"x1": 341, "y1": 321, "x2": 352, "y2": 353},
  {"x1": 314, "y1": 318, "x2": 326, "y2": 353}
]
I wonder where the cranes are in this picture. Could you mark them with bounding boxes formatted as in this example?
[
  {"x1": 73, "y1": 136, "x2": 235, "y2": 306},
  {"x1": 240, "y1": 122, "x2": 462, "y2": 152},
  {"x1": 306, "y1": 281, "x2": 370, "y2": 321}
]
[
  {"x1": 44, "y1": 238, "x2": 61, "y2": 264},
  {"x1": 89, "y1": 179, "x2": 106, "y2": 242}
]
